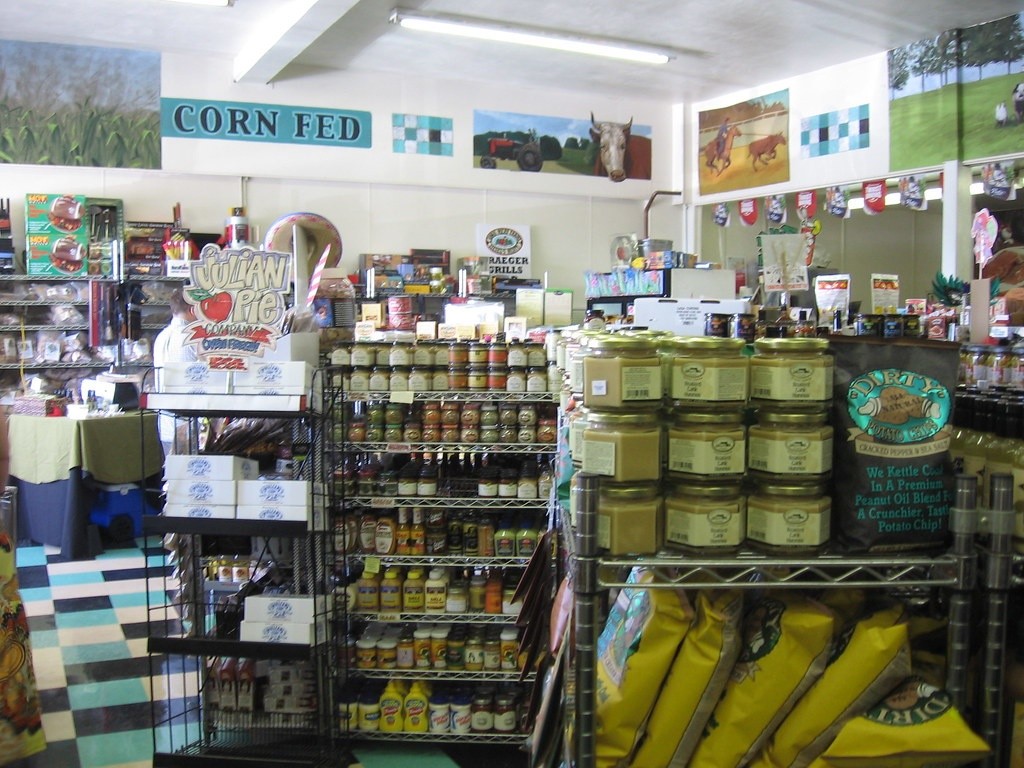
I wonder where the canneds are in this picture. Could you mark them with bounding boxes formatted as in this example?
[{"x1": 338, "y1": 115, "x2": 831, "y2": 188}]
[
  {"x1": 853, "y1": 313, "x2": 921, "y2": 338},
  {"x1": 555, "y1": 309, "x2": 835, "y2": 559},
  {"x1": 329, "y1": 293, "x2": 563, "y2": 736},
  {"x1": 218, "y1": 559, "x2": 262, "y2": 583}
]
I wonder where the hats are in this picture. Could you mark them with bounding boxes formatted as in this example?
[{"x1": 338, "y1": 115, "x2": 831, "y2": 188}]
[{"x1": 263, "y1": 213, "x2": 342, "y2": 283}]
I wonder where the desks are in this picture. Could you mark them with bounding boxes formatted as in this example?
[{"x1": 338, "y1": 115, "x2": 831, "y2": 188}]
[{"x1": 7, "y1": 409, "x2": 161, "y2": 561}]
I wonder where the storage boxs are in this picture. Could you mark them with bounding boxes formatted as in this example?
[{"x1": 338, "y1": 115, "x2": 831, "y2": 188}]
[
  {"x1": 358, "y1": 253, "x2": 430, "y2": 296},
  {"x1": 905, "y1": 298, "x2": 957, "y2": 339},
  {"x1": 157, "y1": 454, "x2": 329, "y2": 533},
  {"x1": 141, "y1": 363, "x2": 323, "y2": 415},
  {"x1": 24, "y1": 195, "x2": 198, "y2": 280},
  {"x1": 240, "y1": 593, "x2": 332, "y2": 646},
  {"x1": 648, "y1": 251, "x2": 698, "y2": 269},
  {"x1": 15, "y1": 396, "x2": 69, "y2": 418}
]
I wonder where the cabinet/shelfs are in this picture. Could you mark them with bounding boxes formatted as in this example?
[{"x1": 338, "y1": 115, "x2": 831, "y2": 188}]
[
  {"x1": 1, "y1": 275, "x2": 192, "y2": 389},
  {"x1": 558, "y1": 469, "x2": 1023, "y2": 768},
  {"x1": 137, "y1": 379, "x2": 356, "y2": 768},
  {"x1": 339, "y1": 392, "x2": 561, "y2": 768}
]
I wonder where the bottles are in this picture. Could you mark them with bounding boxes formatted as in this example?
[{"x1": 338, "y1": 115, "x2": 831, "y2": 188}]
[{"x1": 332, "y1": 453, "x2": 554, "y2": 734}]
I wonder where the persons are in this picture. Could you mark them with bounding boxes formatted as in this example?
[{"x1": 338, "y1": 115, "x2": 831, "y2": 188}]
[
  {"x1": 0, "y1": 409, "x2": 46, "y2": 768},
  {"x1": 153, "y1": 288, "x2": 197, "y2": 456}
]
